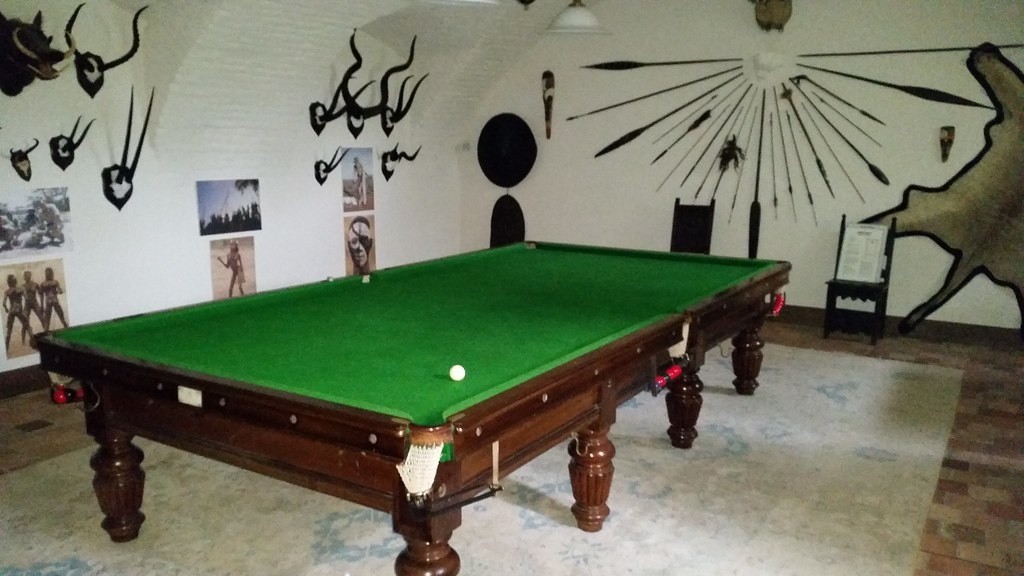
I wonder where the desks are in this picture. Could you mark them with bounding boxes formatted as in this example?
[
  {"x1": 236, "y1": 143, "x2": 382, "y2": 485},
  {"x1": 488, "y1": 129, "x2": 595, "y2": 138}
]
[{"x1": 30, "y1": 241, "x2": 792, "y2": 576}]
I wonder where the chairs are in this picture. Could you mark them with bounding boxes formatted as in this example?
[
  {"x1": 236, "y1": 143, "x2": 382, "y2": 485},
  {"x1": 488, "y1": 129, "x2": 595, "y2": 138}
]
[{"x1": 821, "y1": 213, "x2": 897, "y2": 346}]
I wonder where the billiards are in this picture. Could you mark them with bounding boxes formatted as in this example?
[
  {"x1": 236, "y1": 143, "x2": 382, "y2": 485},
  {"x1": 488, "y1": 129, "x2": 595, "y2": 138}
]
[
  {"x1": 654, "y1": 365, "x2": 682, "y2": 386},
  {"x1": 448, "y1": 363, "x2": 468, "y2": 382},
  {"x1": 53, "y1": 381, "x2": 88, "y2": 404}
]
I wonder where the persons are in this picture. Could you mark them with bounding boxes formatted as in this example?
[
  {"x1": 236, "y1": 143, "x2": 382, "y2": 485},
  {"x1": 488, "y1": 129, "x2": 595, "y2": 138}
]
[
  {"x1": 198, "y1": 202, "x2": 263, "y2": 298},
  {"x1": 348, "y1": 216, "x2": 374, "y2": 275},
  {"x1": 353, "y1": 158, "x2": 367, "y2": 206},
  {"x1": 0, "y1": 200, "x2": 68, "y2": 351}
]
